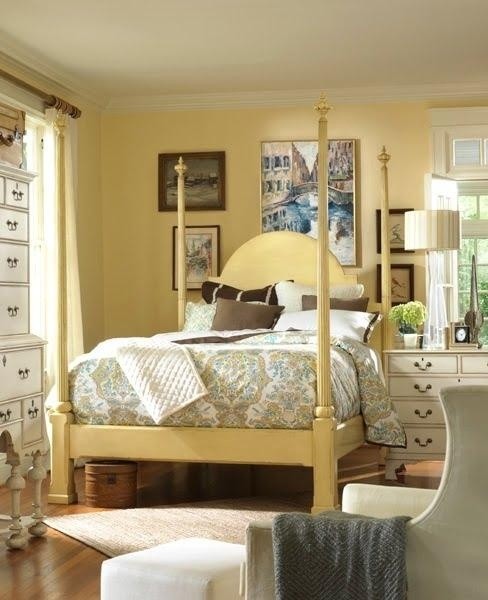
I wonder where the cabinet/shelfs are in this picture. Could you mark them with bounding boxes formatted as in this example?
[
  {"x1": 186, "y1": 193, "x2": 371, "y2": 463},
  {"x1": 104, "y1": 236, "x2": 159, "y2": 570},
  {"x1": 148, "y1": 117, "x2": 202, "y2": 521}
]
[
  {"x1": 0, "y1": 337, "x2": 48, "y2": 489},
  {"x1": 0, "y1": 163, "x2": 36, "y2": 337}
]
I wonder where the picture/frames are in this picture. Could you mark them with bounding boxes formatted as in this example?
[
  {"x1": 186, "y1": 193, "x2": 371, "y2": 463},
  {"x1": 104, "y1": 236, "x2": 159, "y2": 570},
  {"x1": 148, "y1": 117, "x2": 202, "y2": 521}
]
[
  {"x1": 447, "y1": 321, "x2": 479, "y2": 347},
  {"x1": 157, "y1": 151, "x2": 228, "y2": 213},
  {"x1": 375, "y1": 209, "x2": 417, "y2": 254},
  {"x1": 376, "y1": 263, "x2": 415, "y2": 307},
  {"x1": 259, "y1": 140, "x2": 359, "y2": 269},
  {"x1": 171, "y1": 225, "x2": 221, "y2": 292}
]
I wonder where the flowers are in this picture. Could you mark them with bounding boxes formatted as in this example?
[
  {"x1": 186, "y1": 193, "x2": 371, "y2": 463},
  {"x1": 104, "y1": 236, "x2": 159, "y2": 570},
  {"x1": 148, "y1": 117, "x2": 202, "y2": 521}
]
[{"x1": 389, "y1": 302, "x2": 427, "y2": 332}]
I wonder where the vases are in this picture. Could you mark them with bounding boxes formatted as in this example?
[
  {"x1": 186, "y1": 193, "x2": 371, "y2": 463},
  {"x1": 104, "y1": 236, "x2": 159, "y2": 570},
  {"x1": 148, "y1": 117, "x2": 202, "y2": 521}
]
[{"x1": 403, "y1": 334, "x2": 419, "y2": 349}]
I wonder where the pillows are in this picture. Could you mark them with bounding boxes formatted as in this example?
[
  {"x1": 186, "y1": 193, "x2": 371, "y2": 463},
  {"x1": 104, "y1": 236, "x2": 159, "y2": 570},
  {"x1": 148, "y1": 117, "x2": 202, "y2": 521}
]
[
  {"x1": 302, "y1": 294, "x2": 370, "y2": 312},
  {"x1": 202, "y1": 281, "x2": 295, "y2": 306},
  {"x1": 183, "y1": 300, "x2": 270, "y2": 332},
  {"x1": 275, "y1": 281, "x2": 364, "y2": 309},
  {"x1": 271, "y1": 309, "x2": 379, "y2": 343},
  {"x1": 211, "y1": 296, "x2": 286, "y2": 331}
]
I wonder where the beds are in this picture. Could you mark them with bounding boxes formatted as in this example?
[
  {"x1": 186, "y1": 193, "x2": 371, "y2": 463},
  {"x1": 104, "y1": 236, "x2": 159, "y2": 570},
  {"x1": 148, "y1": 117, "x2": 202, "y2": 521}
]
[{"x1": 43, "y1": 89, "x2": 391, "y2": 516}]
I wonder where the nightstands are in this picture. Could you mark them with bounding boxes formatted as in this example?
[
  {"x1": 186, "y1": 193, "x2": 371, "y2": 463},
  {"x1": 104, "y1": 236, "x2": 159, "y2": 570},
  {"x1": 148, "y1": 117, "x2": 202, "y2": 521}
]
[{"x1": 380, "y1": 345, "x2": 488, "y2": 481}]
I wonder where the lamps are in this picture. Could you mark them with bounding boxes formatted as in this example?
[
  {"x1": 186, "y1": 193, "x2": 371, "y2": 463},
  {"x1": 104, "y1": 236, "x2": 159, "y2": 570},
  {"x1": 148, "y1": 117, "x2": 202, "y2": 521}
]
[{"x1": 403, "y1": 207, "x2": 460, "y2": 350}]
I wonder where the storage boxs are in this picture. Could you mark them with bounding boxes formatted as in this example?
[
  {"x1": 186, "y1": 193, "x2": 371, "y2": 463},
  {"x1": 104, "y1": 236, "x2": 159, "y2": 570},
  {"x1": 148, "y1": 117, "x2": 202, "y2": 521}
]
[
  {"x1": 0, "y1": 128, "x2": 25, "y2": 167},
  {"x1": 0, "y1": 100, "x2": 27, "y2": 136}
]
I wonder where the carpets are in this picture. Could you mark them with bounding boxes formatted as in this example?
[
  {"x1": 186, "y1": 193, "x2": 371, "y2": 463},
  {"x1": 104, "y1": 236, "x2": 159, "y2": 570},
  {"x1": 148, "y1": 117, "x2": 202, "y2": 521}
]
[{"x1": 42, "y1": 494, "x2": 310, "y2": 559}]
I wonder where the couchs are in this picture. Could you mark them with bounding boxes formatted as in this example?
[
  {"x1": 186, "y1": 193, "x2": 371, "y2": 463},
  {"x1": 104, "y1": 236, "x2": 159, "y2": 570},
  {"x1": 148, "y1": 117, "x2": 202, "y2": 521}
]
[{"x1": 100, "y1": 386, "x2": 485, "y2": 600}]
